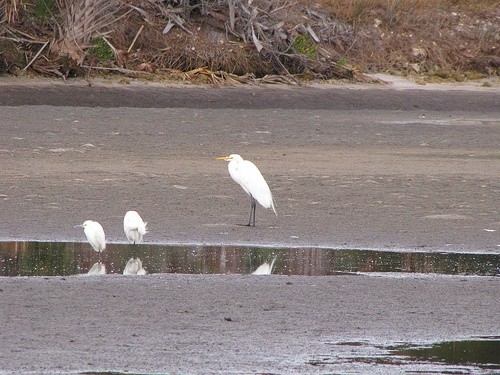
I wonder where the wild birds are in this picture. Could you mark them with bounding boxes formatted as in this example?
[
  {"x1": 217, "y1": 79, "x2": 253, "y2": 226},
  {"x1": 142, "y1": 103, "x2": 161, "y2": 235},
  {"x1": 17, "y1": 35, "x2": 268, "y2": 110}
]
[
  {"x1": 123, "y1": 210, "x2": 150, "y2": 248},
  {"x1": 73, "y1": 219, "x2": 107, "y2": 258},
  {"x1": 216, "y1": 153, "x2": 279, "y2": 228}
]
